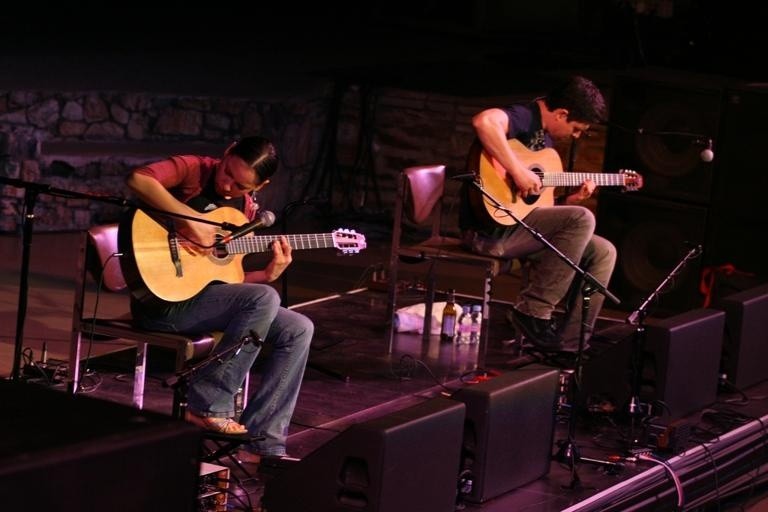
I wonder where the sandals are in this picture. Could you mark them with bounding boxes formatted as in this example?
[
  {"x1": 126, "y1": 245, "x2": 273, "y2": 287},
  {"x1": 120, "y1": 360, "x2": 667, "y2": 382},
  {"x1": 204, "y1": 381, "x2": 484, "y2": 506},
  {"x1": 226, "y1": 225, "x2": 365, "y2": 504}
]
[
  {"x1": 270, "y1": 455, "x2": 302, "y2": 462},
  {"x1": 184, "y1": 410, "x2": 250, "y2": 436}
]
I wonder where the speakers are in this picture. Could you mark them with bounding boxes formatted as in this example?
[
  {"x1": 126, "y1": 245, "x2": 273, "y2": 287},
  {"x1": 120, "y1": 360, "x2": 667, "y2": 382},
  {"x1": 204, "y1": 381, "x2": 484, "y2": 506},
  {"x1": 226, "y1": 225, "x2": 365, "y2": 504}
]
[
  {"x1": 259, "y1": 398, "x2": 465, "y2": 512},
  {"x1": 721, "y1": 284, "x2": 768, "y2": 393},
  {"x1": 450, "y1": 363, "x2": 561, "y2": 503},
  {"x1": 582, "y1": 309, "x2": 725, "y2": 427},
  {"x1": 602, "y1": 73, "x2": 768, "y2": 207},
  {"x1": 0, "y1": 378, "x2": 202, "y2": 512},
  {"x1": 598, "y1": 189, "x2": 768, "y2": 318}
]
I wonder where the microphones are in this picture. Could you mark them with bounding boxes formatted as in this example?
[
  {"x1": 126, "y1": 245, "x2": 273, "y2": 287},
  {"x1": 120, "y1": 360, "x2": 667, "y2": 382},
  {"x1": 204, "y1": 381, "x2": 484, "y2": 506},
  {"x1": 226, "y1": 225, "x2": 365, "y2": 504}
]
[
  {"x1": 214, "y1": 210, "x2": 276, "y2": 247},
  {"x1": 700, "y1": 138, "x2": 714, "y2": 163},
  {"x1": 447, "y1": 170, "x2": 477, "y2": 180}
]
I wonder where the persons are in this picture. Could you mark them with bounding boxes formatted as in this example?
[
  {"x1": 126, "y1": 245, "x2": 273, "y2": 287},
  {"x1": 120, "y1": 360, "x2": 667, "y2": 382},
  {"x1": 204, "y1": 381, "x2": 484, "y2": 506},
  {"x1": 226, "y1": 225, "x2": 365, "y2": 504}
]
[
  {"x1": 459, "y1": 72, "x2": 617, "y2": 364},
  {"x1": 123, "y1": 137, "x2": 314, "y2": 466}
]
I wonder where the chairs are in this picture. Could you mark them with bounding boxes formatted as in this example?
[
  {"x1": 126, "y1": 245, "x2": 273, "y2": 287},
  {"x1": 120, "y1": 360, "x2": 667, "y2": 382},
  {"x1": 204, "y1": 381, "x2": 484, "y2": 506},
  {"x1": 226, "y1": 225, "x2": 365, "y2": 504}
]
[
  {"x1": 387, "y1": 165, "x2": 532, "y2": 370},
  {"x1": 67, "y1": 222, "x2": 249, "y2": 421}
]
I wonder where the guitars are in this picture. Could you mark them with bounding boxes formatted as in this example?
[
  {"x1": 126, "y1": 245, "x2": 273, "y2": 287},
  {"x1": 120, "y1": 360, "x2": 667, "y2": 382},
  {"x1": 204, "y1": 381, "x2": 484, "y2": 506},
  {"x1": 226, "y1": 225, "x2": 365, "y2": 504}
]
[
  {"x1": 465, "y1": 135, "x2": 643, "y2": 226},
  {"x1": 117, "y1": 203, "x2": 366, "y2": 303}
]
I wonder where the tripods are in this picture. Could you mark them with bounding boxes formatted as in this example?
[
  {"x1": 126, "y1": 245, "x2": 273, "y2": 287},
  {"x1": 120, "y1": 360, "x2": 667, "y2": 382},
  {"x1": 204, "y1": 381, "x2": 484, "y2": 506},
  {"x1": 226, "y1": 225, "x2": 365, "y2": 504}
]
[
  {"x1": 466, "y1": 182, "x2": 625, "y2": 488},
  {"x1": 581, "y1": 245, "x2": 702, "y2": 458}
]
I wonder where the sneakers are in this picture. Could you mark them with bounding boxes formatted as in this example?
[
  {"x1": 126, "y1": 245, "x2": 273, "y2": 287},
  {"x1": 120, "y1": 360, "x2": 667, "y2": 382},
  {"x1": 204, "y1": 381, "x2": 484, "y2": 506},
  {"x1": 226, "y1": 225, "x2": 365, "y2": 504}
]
[
  {"x1": 511, "y1": 307, "x2": 565, "y2": 353},
  {"x1": 543, "y1": 349, "x2": 578, "y2": 375}
]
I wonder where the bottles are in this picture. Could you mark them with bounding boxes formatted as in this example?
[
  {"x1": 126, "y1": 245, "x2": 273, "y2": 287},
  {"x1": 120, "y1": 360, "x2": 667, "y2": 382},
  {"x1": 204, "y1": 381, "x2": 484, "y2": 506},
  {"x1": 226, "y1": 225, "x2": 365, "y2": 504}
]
[{"x1": 441, "y1": 288, "x2": 482, "y2": 344}]
